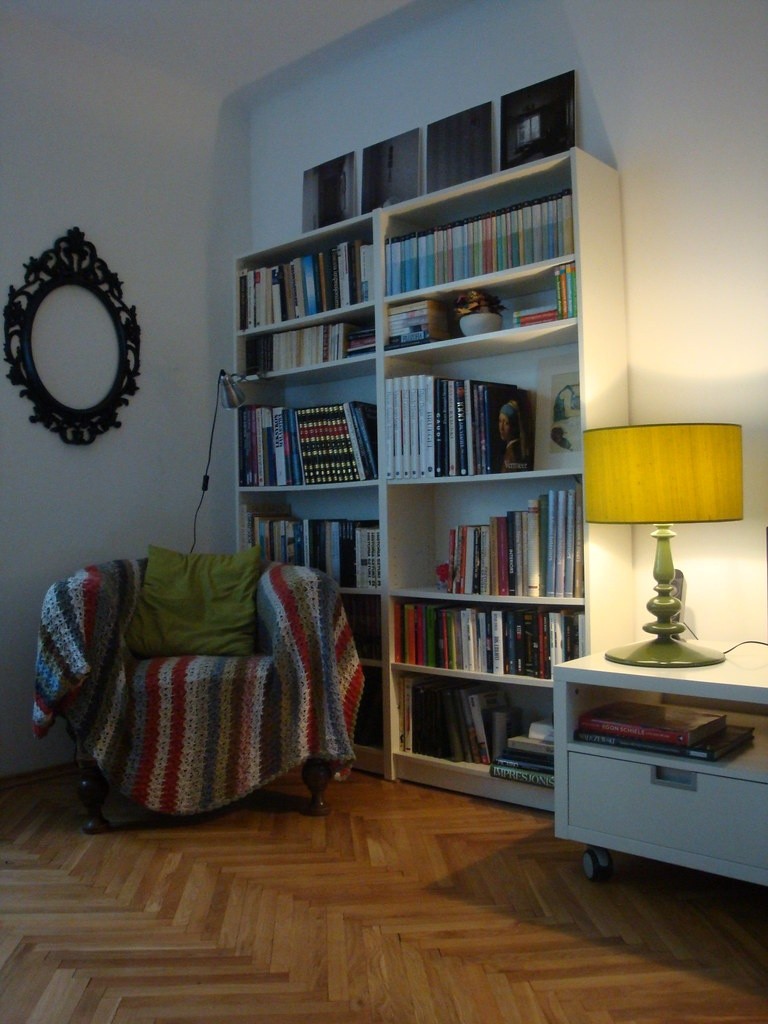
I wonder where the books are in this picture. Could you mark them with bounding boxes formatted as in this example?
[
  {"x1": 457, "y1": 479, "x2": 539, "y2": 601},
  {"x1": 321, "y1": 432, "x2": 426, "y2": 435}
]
[
  {"x1": 240, "y1": 239, "x2": 374, "y2": 331},
  {"x1": 241, "y1": 504, "x2": 381, "y2": 589},
  {"x1": 490, "y1": 718, "x2": 554, "y2": 788},
  {"x1": 384, "y1": 299, "x2": 450, "y2": 351},
  {"x1": 239, "y1": 401, "x2": 379, "y2": 486},
  {"x1": 385, "y1": 375, "x2": 532, "y2": 479},
  {"x1": 574, "y1": 700, "x2": 754, "y2": 761},
  {"x1": 449, "y1": 486, "x2": 584, "y2": 600},
  {"x1": 384, "y1": 188, "x2": 575, "y2": 298},
  {"x1": 394, "y1": 602, "x2": 585, "y2": 679},
  {"x1": 398, "y1": 675, "x2": 514, "y2": 763},
  {"x1": 342, "y1": 596, "x2": 381, "y2": 660},
  {"x1": 245, "y1": 322, "x2": 376, "y2": 374}
]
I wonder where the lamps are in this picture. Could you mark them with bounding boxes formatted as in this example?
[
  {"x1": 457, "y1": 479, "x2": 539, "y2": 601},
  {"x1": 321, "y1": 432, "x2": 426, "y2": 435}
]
[
  {"x1": 582, "y1": 422, "x2": 745, "y2": 668},
  {"x1": 220, "y1": 370, "x2": 246, "y2": 412}
]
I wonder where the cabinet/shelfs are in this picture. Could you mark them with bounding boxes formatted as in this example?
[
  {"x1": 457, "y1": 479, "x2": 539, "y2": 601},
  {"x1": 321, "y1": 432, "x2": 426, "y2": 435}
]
[
  {"x1": 553, "y1": 643, "x2": 768, "y2": 891},
  {"x1": 232, "y1": 146, "x2": 632, "y2": 812}
]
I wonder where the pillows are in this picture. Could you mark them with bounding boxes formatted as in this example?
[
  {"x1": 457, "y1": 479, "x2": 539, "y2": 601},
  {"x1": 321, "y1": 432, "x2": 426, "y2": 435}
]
[{"x1": 126, "y1": 544, "x2": 261, "y2": 656}]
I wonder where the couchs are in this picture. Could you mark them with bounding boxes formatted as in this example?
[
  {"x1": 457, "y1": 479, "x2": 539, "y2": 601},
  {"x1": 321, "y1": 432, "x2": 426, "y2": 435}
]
[{"x1": 32, "y1": 557, "x2": 366, "y2": 835}]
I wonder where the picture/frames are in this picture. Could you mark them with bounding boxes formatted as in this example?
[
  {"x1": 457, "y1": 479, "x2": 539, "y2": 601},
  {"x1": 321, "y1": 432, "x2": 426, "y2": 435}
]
[{"x1": 533, "y1": 353, "x2": 584, "y2": 470}]
